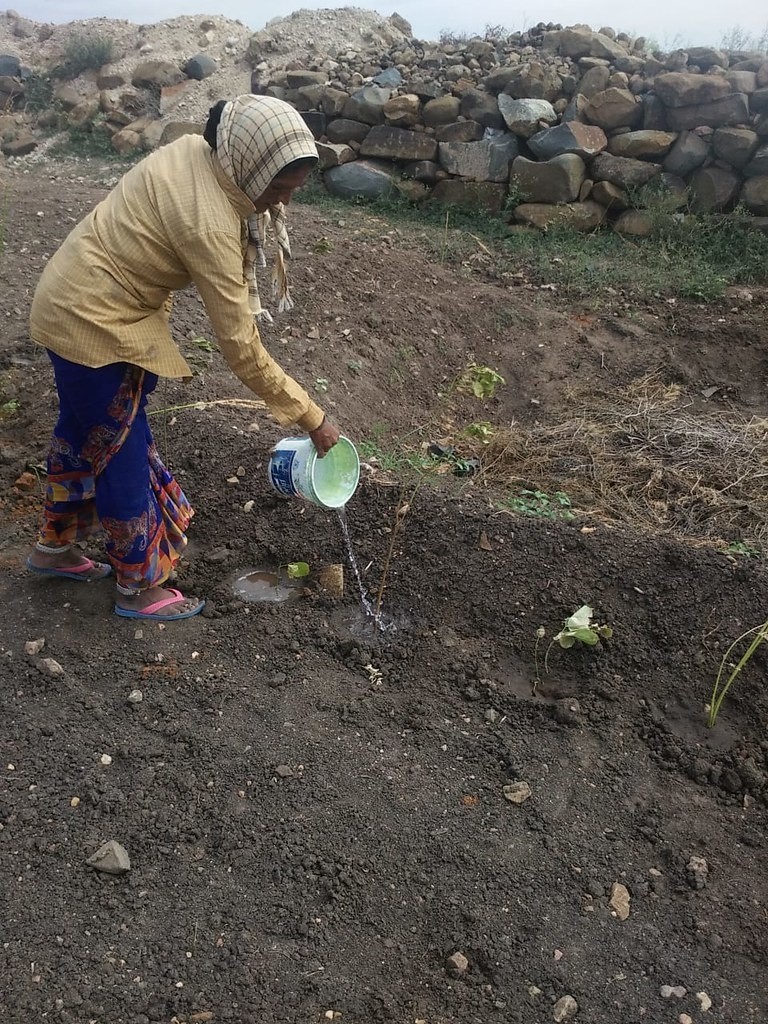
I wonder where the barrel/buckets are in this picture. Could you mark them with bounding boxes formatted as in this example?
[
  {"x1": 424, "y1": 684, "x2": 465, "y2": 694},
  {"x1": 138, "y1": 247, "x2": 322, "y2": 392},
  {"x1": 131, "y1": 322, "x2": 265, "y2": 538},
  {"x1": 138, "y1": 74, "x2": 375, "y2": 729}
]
[{"x1": 268, "y1": 432, "x2": 361, "y2": 509}]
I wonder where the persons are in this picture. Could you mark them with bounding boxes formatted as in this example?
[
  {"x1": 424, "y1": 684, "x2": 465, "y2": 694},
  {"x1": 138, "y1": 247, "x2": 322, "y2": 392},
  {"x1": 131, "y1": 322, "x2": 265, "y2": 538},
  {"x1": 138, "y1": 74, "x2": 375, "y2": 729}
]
[{"x1": 25, "y1": 92, "x2": 320, "y2": 618}]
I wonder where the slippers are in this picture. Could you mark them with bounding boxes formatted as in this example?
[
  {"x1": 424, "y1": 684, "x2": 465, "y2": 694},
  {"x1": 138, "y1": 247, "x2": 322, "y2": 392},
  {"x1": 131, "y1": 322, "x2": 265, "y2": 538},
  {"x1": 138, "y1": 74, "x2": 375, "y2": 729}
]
[
  {"x1": 114, "y1": 589, "x2": 205, "y2": 621},
  {"x1": 28, "y1": 555, "x2": 112, "y2": 582}
]
